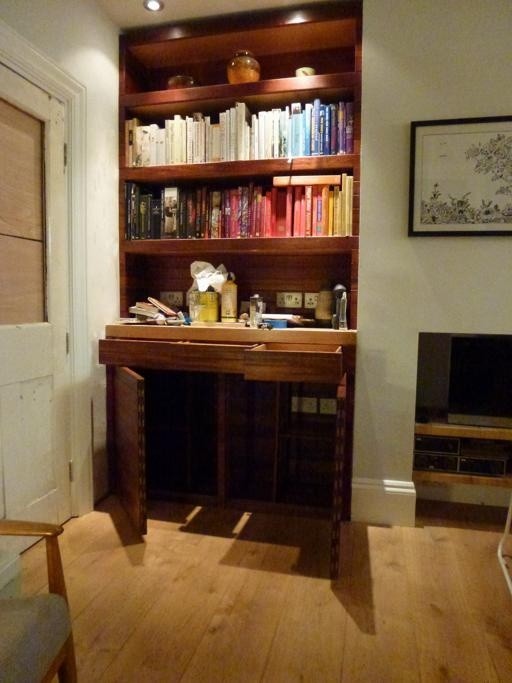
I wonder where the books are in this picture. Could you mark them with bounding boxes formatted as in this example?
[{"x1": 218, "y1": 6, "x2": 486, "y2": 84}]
[
  {"x1": 125, "y1": 98, "x2": 354, "y2": 167},
  {"x1": 124, "y1": 293, "x2": 182, "y2": 326},
  {"x1": 126, "y1": 172, "x2": 354, "y2": 239}
]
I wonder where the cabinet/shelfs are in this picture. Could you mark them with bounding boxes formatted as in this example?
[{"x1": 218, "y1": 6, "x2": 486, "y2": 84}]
[
  {"x1": 97, "y1": 5, "x2": 363, "y2": 538},
  {"x1": 413, "y1": 422, "x2": 512, "y2": 522}
]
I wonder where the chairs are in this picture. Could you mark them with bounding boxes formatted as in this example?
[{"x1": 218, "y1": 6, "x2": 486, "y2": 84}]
[{"x1": 2, "y1": 520, "x2": 80, "y2": 682}]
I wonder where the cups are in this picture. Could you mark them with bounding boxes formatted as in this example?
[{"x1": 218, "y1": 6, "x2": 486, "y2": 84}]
[{"x1": 249, "y1": 293, "x2": 262, "y2": 328}]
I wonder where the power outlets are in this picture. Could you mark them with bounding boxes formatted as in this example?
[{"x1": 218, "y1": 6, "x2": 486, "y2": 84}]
[
  {"x1": 155, "y1": 292, "x2": 182, "y2": 307},
  {"x1": 275, "y1": 291, "x2": 301, "y2": 308},
  {"x1": 305, "y1": 291, "x2": 320, "y2": 307}
]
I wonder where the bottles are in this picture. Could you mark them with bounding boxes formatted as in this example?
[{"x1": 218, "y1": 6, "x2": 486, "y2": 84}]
[
  {"x1": 314, "y1": 281, "x2": 338, "y2": 327},
  {"x1": 220, "y1": 272, "x2": 237, "y2": 322}
]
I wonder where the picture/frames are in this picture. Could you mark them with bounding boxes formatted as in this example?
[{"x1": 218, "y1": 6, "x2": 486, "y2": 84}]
[{"x1": 409, "y1": 115, "x2": 511, "y2": 238}]
